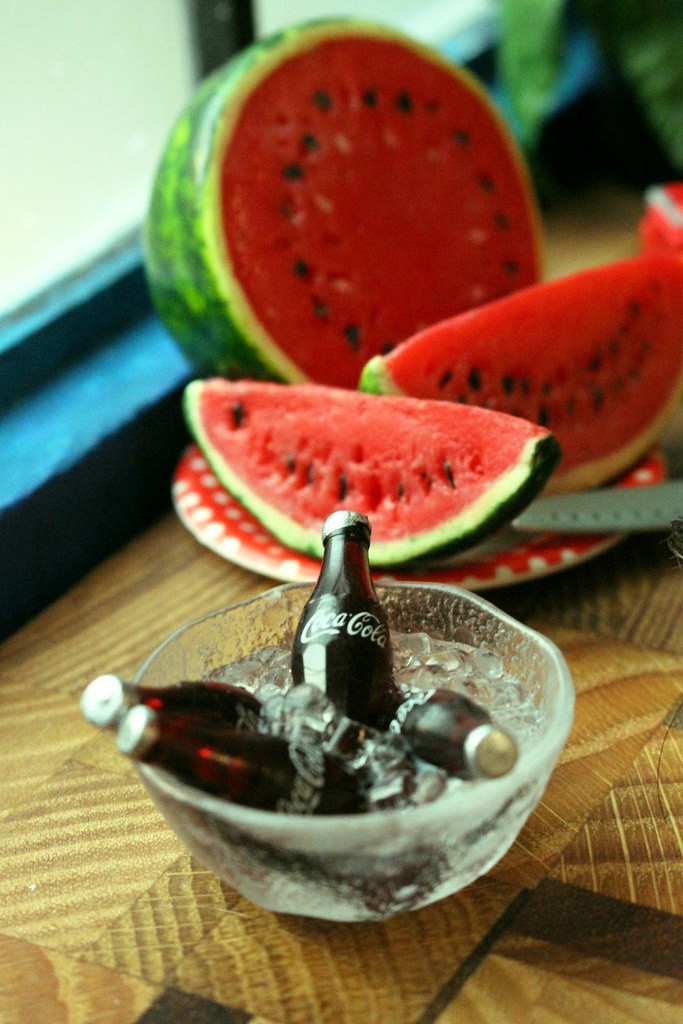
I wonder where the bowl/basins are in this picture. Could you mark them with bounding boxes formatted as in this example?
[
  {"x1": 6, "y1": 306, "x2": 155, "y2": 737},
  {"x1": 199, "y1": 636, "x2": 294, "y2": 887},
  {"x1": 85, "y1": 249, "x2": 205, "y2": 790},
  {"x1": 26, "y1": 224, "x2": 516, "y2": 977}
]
[{"x1": 127, "y1": 578, "x2": 578, "y2": 921}]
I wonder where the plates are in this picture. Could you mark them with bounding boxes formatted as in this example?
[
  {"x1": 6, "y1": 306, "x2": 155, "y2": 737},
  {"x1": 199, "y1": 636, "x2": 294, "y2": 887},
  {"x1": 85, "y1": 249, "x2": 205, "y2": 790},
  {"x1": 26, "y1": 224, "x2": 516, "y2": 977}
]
[{"x1": 169, "y1": 441, "x2": 665, "y2": 590}]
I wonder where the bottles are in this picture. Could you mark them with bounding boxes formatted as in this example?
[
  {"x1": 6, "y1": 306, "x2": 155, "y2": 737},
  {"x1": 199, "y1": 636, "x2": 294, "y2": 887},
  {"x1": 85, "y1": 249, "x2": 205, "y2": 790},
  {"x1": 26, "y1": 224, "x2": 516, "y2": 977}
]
[
  {"x1": 116, "y1": 707, "x2": 449, "y2": 911},
  {"x1": 83, "y1": 673, "x2": 265, "y2": 734},
  {"x1": 292, "y1": 507, "x2": 395, "y2": 725},
  {"x1": 395, "y1": 680, "x2": 519, "y2": 779}
]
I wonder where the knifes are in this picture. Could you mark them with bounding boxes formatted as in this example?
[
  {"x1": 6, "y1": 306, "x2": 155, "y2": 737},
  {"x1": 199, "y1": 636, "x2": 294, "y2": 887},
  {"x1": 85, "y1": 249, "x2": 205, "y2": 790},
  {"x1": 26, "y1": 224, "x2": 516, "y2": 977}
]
[{"x1": 506, "y1": 481, "x2": 683, "y2": 532}]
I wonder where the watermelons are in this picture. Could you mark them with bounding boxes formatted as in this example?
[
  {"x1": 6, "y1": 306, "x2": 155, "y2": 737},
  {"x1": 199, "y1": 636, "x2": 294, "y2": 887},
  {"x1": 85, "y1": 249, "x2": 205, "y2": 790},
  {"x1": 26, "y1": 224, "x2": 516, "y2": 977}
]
[{"x1": 142, "y1": 10, "x2": 682, "y2": 570}]
